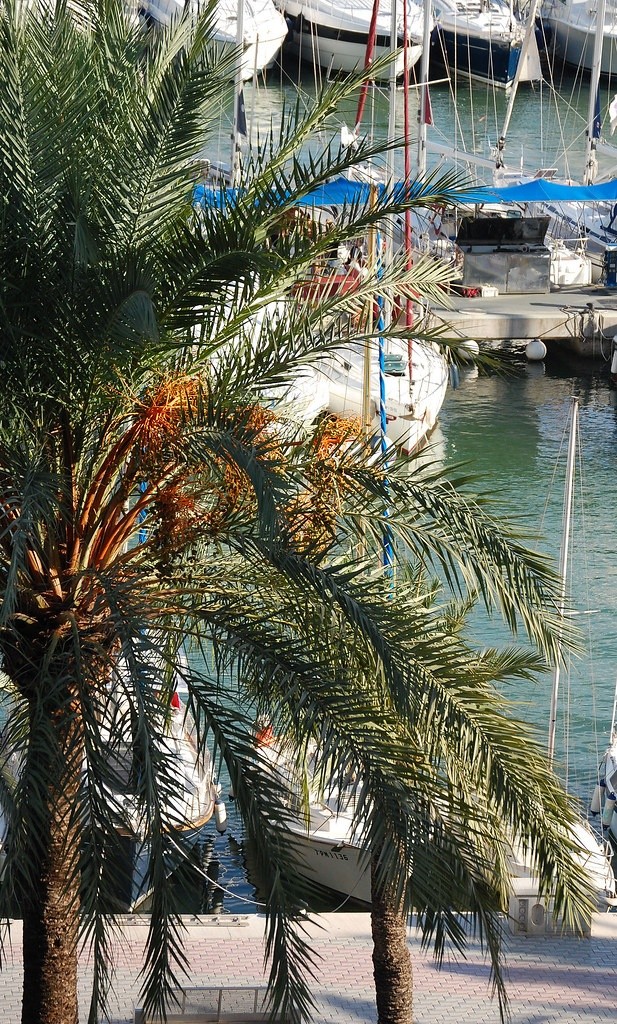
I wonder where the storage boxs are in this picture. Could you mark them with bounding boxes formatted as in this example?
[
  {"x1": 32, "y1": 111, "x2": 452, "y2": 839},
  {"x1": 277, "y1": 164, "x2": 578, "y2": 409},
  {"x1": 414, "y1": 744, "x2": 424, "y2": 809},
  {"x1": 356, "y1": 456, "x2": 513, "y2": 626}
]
[{"x1": 476, "y1": 286, "x2": 498, "y2": 297}]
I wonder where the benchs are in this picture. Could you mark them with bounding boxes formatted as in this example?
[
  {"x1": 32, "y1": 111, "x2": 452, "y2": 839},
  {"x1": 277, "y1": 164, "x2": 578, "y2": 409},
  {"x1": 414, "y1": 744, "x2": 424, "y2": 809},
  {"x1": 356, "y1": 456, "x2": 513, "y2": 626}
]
[
  {"x1": 132, "y1": 986, "x2": 301, "y2": 1024},
  {"x1": 447, "y1": 216, "x2": 551, "y2": 252}
]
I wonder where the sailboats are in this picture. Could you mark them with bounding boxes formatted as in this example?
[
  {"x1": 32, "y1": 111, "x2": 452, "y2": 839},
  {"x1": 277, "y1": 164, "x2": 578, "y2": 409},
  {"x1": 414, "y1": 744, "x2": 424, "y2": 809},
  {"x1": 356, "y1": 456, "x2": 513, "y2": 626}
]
[{"x1": 0, "y1": 0, "x2": 616, "y2": 910}]
